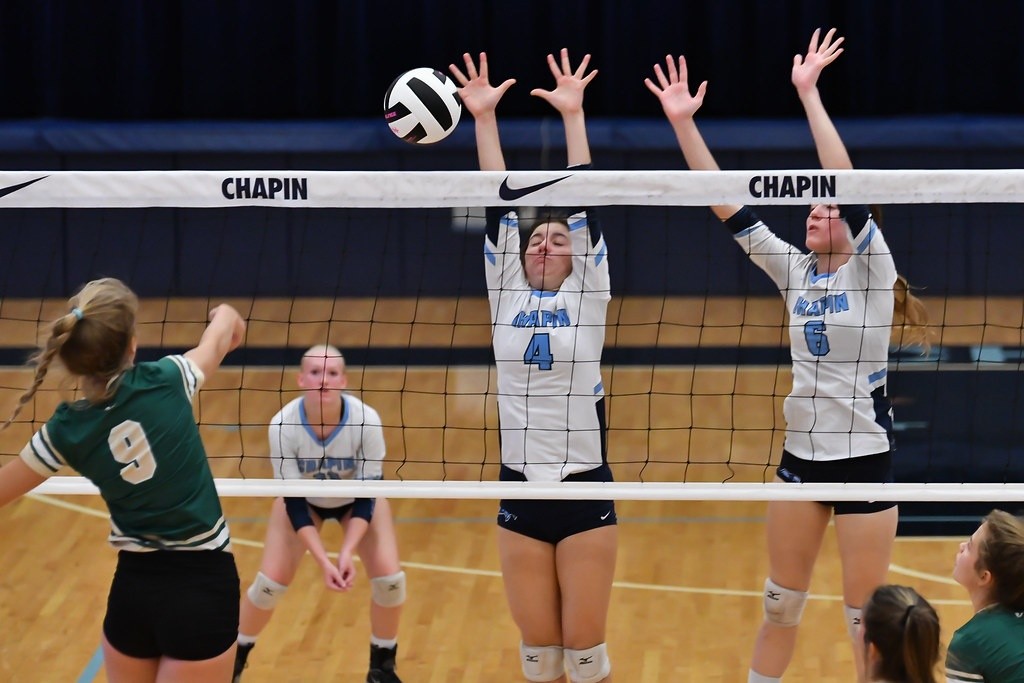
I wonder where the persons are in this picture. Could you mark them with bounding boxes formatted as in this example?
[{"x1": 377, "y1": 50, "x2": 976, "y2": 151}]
[
  {"x1": 449, "y1": 47, "x2": 619, "y2": 683},
  {"x1": 943, "y1": 508, "x2": 1024, "y2": 683},
  {"x1": 232, "y1": 346, "x2": 407, "y2": 682},
  {"x1": 0, "y1": 277, "x2": 246, "y2": 681},
  {"x1": 855, "y1": 585, "x2": 942, "y2": 682},
  {"x1": 645, "y1": 27, "x2": 935, "y2": 683}
]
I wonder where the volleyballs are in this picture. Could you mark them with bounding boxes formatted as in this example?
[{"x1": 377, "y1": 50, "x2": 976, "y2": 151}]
[{"x1": 383, "y1": 66, "x2": 462, "y2": 145}]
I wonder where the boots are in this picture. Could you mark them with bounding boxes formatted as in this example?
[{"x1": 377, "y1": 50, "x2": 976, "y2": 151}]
[
  {"x1": 364, "y1": 642, "x2": 402, "y2": 683},
  {"x1": 233, "y1": 640, "x2": 256, "y2": 682}
]
